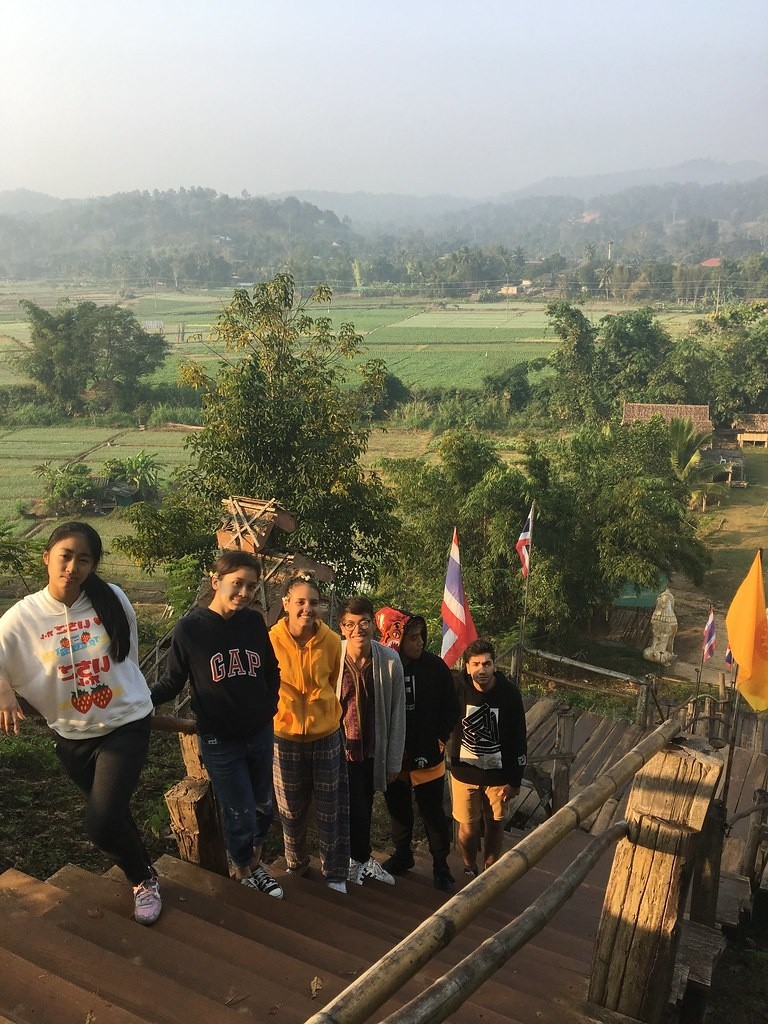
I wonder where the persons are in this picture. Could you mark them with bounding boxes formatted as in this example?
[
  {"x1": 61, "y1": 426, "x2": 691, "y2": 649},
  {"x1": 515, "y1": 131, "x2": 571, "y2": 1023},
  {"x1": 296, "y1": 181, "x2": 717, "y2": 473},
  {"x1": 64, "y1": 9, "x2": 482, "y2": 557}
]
[
  {"x1": 330, "y1": 595, "x2": 395, "y2": 887},
  {"x1": 0, "y1": 520, "x2": 162, "y2": 924},
  {"x1": 376, "y1": 605, "x2": 455, "y2": 895},
  {"x1": 149, "y1": 551, "x2": 284, "y2": 902},
  {"x1": 449, "y1": 640, "x2": 527, "y2": 877},
  {"x1": 268, "y1": 578, "x2": 351, "y2": 893}
]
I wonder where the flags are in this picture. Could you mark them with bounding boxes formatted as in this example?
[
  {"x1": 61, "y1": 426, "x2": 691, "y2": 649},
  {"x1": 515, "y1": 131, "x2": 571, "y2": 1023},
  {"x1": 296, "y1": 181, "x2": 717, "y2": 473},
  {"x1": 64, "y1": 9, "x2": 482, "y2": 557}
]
[
  {"x1": 703, "y1": 606, "x2": 716, "y2": 664},
  {"x1": 515, "y1": 504, "x2": 533, "y2": 580},
  {"x1": 724, "y1": 550, "x2": 768, "y2": 711},
  {"x1": 442, "y1": 526, "x2": 479, "y2": 669}
]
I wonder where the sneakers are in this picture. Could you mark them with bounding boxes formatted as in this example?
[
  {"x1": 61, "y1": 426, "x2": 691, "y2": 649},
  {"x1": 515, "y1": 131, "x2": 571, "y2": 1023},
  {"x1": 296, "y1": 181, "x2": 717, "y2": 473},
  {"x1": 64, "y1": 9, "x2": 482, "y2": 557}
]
[
  {"x1": 432, "y1": 866, "x2": 456, "y2": 896},
  {"x1": 230, "y1": 865, "x2": 283, "y2": 899},
  {"x1": 382, "y1": 853, "x2": 408, "y2": 876},
  {"x1": 327, "y1": 880, "x2": 347, "y2": 895},
  {"x1": 463, "y1": 864, "x2": 486, "y2": 879},
  {"x1": 283, "y1": 863, "x2": 309, "y2": 877},
  {"x1": 132, "y1": 864, "x2": 162, "y2": 925},
  {"x1": 348, "y1": 856, "x2": 395, "y2": 887}
]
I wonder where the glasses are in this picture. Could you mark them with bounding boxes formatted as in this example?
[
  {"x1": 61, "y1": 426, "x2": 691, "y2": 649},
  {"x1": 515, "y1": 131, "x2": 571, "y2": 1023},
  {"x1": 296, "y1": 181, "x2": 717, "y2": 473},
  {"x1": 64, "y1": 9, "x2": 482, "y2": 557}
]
[{"x1": 341, "y1": 619, "x2": 373, "y2": 631}]
